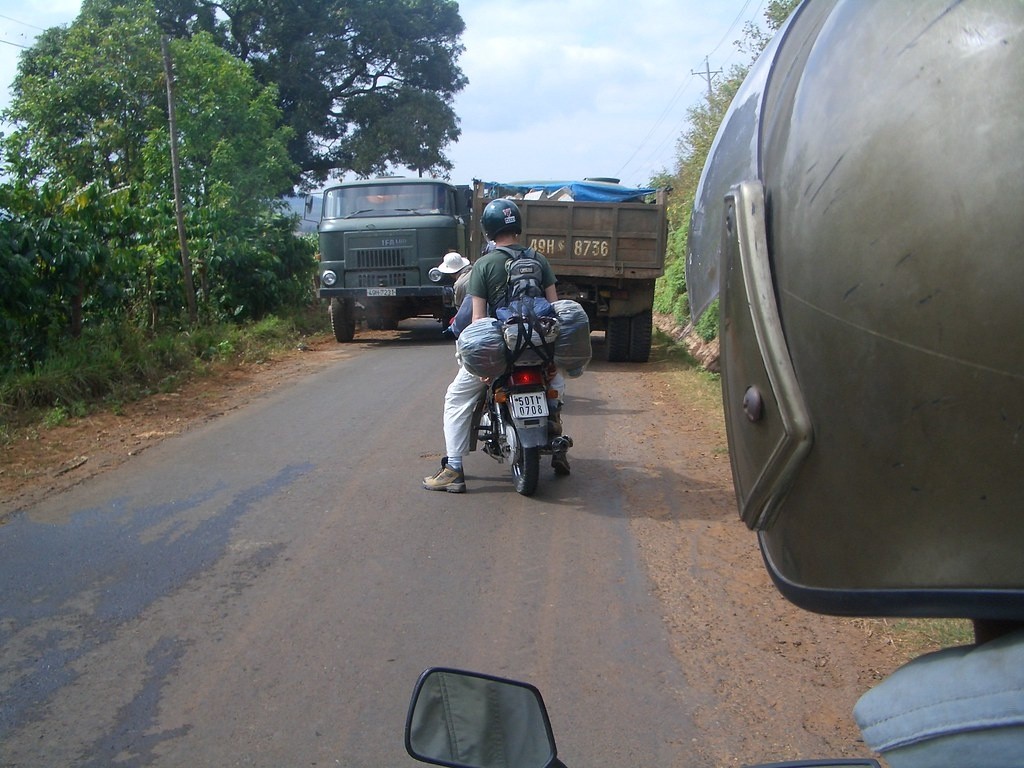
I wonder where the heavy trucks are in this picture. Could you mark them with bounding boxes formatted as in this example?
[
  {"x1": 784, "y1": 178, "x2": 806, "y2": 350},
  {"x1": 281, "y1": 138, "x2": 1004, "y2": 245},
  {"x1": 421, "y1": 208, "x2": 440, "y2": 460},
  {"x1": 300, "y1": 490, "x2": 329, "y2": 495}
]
[
  {"x1": 467, "y1": 176, "x2": 668, "y2": 364},
  {"x1": 303, "y1": 175, "x2": 473, "y2": 344}
]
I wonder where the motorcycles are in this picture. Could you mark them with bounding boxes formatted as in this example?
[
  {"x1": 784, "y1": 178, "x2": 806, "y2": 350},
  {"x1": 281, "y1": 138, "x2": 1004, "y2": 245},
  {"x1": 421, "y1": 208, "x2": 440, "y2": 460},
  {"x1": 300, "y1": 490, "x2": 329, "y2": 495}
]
[{"x1": 441, "y1": 284, "x2": 575, "y2": 497}]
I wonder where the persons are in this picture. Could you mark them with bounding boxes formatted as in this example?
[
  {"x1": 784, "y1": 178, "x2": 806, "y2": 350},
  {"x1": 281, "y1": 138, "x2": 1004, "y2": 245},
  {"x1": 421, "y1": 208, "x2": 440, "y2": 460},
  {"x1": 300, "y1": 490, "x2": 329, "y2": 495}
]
[
  {"x1": 422, "y1": 199, "x2": 571, "y2": 494},
  {"x1": 702, "y1": 0, "x2": 1024, "y2": 767},
  {"x1": 410, "y1": 672, "x2": 553, "y2": 768}
]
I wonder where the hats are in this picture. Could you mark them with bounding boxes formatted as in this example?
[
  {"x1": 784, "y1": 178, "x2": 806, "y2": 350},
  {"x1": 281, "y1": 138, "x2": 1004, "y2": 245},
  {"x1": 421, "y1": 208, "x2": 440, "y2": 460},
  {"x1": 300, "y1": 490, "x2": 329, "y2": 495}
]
[{"x1": 438, "y1": 252, "x2": 470, "y2": 273}]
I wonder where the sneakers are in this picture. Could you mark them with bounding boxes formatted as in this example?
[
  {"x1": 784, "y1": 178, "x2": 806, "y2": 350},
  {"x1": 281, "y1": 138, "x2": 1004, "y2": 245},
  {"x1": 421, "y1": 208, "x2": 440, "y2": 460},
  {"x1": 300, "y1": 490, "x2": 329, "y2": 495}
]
[
  {"x1": 551, "y1": 449, "x2": 570, "y2": 477},
  {"x1": 423, "y1": 456, "x2": 466, "y2": 492}
]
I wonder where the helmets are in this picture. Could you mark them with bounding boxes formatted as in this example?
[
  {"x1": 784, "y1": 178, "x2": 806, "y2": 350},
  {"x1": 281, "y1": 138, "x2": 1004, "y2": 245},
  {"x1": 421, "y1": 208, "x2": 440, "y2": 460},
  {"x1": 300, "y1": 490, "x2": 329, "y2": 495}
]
[
  {"x1": 720, "y1": 0, "x2": 1024, "y2": 616},
  {"x1": 480, "y1": 198, "x2": 522, "y2": 241}
]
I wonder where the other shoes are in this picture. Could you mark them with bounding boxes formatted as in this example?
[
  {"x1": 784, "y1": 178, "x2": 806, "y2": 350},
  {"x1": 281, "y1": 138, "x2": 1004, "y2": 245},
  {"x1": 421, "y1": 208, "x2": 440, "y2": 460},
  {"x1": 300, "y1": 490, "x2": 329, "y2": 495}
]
[{"x1": 548, "y1": 411, "x2": 562, "y2": 434}]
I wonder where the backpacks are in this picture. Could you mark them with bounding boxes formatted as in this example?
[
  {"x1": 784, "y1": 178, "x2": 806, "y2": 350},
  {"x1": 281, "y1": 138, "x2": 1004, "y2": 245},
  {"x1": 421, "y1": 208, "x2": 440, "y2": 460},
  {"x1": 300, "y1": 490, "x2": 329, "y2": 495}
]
[{"x1": 482, "y1": 245, "x2": 545, "y2": 307}]
